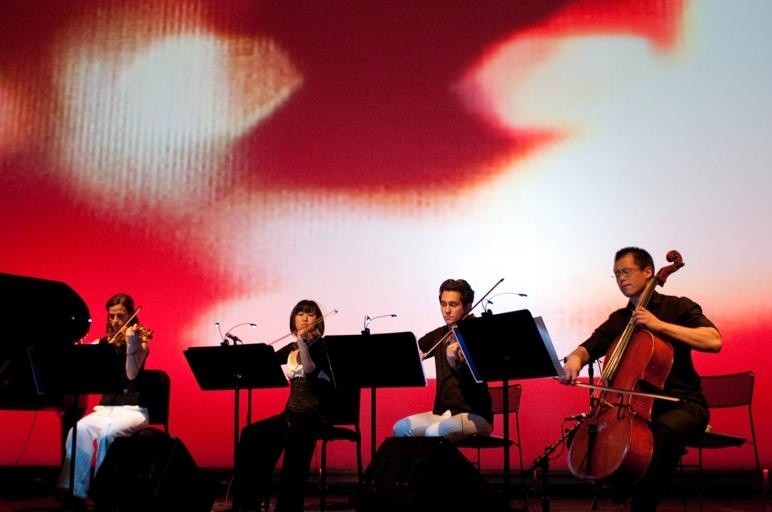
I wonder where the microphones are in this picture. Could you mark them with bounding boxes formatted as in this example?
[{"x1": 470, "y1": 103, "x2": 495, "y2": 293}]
[
  {"x1": 225, "y1": 333, "x2": 242, "y2": 342},
  {"x1": 563, "y1": 412, "x2": 587, "y2": 421}
]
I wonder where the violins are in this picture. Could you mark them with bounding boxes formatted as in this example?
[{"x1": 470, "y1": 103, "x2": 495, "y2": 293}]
[
  {"x1": 444, "y1": 321, "x2": 465, "y2": 363},
  {"x1": 299, "y1": 329, "x2": 321, "y2": 346},
  {"x1": 108, "y1": 324, "x2": 154, "y2": 346}
]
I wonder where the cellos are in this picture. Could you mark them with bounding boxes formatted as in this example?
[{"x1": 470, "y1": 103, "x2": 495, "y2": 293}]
[{"x1": 569, "y1": 250, "x2": 683, "y2": 477}]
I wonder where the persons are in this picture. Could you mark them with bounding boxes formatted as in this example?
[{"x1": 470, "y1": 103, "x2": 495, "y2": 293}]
[
  {"x1": 55, "y1": 295, "x2": 150, "y2": 511},
  {"x1": 232, "y1": 300, "x2": 359, "y2": 511},
  {"x1": 391, "y1": 279, "x2": 497, "y2": 443},
  {"x1": 559, "y1": 246, "x2": 723, "y2": 512}
]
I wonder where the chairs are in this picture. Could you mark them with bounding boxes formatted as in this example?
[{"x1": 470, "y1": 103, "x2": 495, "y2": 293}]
[
  {"x1": 273, "y1": 375, "x2": 363, "y2": 504},
  {"x1": 431, "y1": 373, "x2": 517, "y2": 486},
  {"x1": 136, "y1": 370, "x2": 173, "y2": 437},
  {"x1": 669, "y1": 374, "x2": 757, "y2": 483}
]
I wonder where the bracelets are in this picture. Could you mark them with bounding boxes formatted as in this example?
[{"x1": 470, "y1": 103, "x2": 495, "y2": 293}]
[{"x1": 126, "y1": 349, "x2": 137, "y2": 356}]
